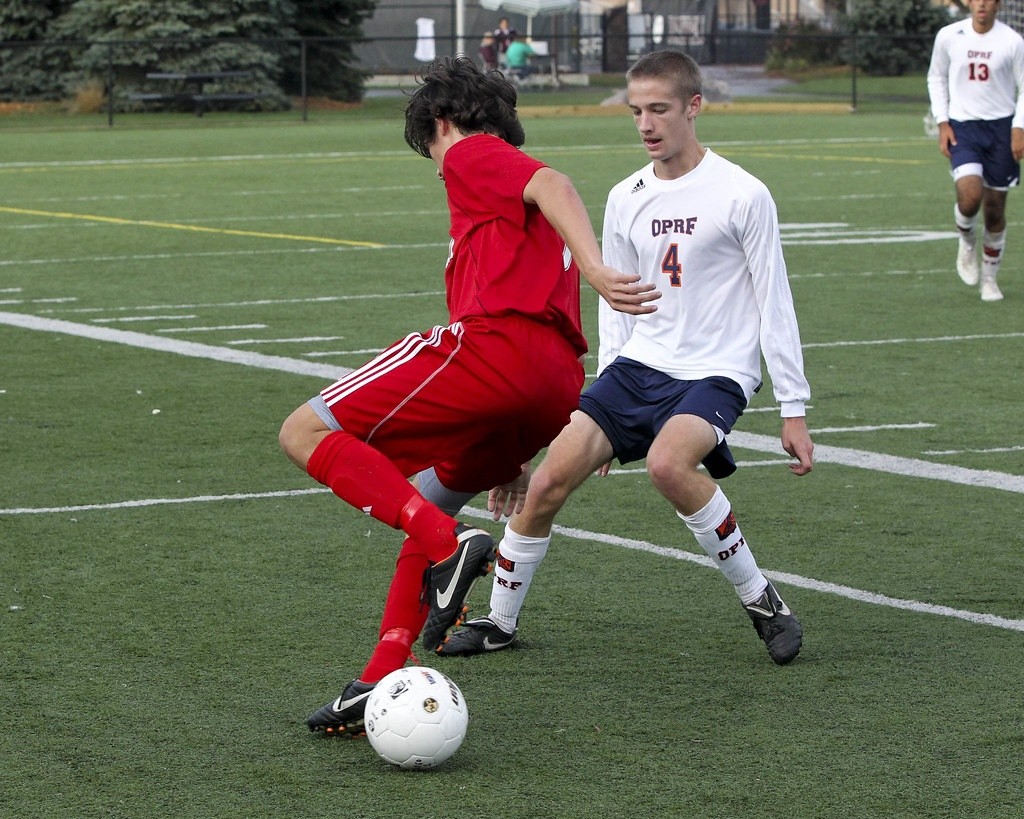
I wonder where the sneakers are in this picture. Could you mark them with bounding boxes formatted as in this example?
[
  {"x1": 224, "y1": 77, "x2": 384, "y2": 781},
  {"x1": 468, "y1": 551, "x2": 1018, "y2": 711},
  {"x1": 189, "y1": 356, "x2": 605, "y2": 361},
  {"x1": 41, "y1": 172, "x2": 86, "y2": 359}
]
[
  {"x1": 740, "y1": 574, "x2": 802, "y2": 666},
  {"x1": 956, "y1": 233, "x2": 980, "y2": 287},
  {"x1": 418, "y1": 522, "x2": 499, "y2": 651},
  {"x1": 436, "y1": 614, "x2": 519, "y2": 655},
  {"x1": 980, "y1": 277, "x2": 1003, "y2": 302},
  {"x1": 306, "y1": 678, "x2": 386, "y2": 738}
]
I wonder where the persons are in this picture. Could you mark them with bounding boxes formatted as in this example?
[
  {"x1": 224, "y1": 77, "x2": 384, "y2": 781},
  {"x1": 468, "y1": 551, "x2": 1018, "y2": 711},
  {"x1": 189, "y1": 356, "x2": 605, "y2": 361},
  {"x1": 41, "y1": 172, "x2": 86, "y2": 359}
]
[
  {"x1": 478, "y1": 15, "x2": 536, "y2": 82},
  {"x1": 432, "y1": 51, "x2": 820, "y2": 665},
  {"x1": 927, "y1": 0, "x2": 1023, "y2": 301},
  {"x1": 275, "y1": 57, "x2": 665, "y2": 741}
]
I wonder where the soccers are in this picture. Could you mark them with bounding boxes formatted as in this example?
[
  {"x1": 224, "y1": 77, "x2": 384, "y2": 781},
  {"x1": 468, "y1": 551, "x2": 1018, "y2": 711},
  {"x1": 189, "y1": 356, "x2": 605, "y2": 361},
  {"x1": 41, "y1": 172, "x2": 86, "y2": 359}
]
[{"x1": 365, "y1": 666, "x2": 468, "y2": 772}]
